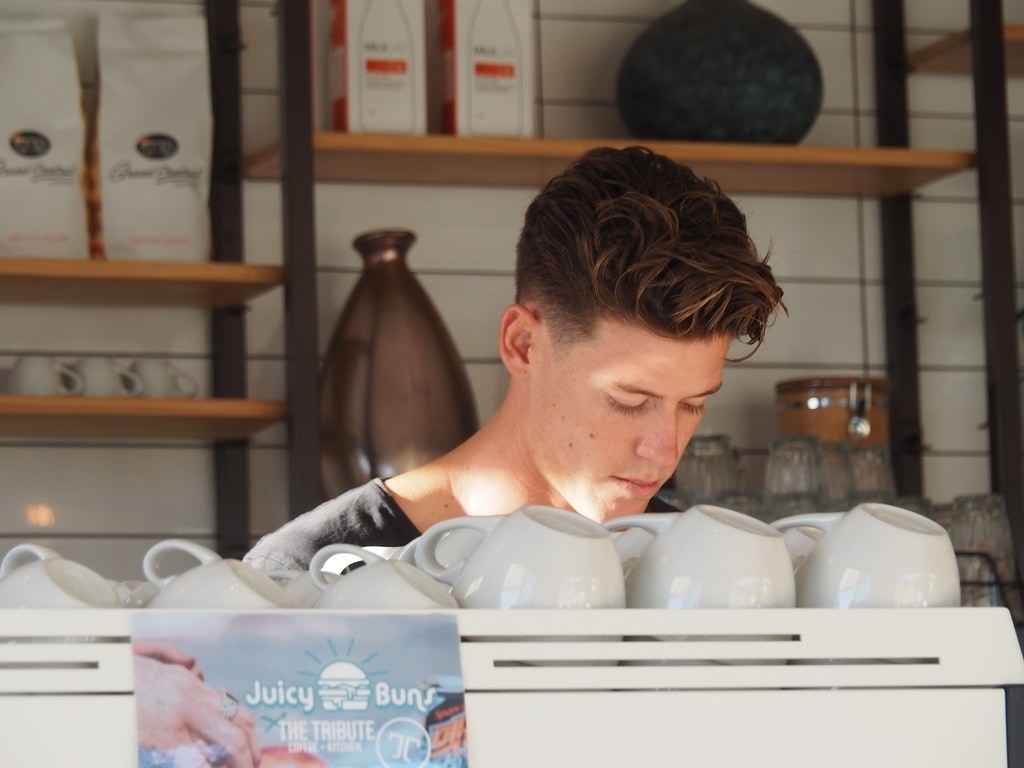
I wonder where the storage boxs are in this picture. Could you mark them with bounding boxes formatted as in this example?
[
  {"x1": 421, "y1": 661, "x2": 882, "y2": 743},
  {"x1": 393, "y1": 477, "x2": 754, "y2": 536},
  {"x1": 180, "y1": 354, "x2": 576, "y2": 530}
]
[
  {"x1": 435, "y1": 0, "x2": 537, "y2": 138},
  {"x1": 329, "y1": 0, "x2": 428, "y2": 135}
]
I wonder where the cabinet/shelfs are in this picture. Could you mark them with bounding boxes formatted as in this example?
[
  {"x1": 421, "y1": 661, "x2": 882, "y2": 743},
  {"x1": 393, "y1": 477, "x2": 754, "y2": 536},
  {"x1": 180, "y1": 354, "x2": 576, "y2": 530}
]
[{"x1": 0, "y1": 0, "x2": 1024, "y2": 628}]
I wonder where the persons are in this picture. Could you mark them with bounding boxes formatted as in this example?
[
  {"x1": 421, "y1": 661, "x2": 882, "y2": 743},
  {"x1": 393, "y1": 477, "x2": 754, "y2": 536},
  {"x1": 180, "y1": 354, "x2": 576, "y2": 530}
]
[
  {"x1": 236, "y1": 145, "x2": 789, "y2": 577},
  {"x1": 132, "y1": 638, "x2": 263, "y2": 768}
]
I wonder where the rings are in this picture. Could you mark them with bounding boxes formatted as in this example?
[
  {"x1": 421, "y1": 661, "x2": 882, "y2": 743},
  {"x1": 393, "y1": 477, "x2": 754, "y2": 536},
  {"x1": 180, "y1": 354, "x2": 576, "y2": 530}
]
[{"x1": 220, "y1": 688, "x2": 239, "y2": 721}]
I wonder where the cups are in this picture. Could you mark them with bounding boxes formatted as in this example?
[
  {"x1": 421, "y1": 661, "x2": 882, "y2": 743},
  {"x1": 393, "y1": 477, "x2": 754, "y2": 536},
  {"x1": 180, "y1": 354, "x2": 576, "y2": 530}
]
[
  {"x1": 308, "y1": 544, "x2": 460, "y2": 610},
  {"x1": 397, "y1": 528, "x2": 484, "y2": 587},
  {"x1": 415, "y1": 504, "x2": 626, "y2": 670},
  {"x1": 906, "y1": 494, "x2": 1021, "y2": 621},
  {"x1": 614, "y1": 523, "x2": 654, "y2": 573},
  {"x1": 677, "y1": 435, "x2": 741, "y2": 504},
  {"x1": 765, "y1": 436, "x2": 896, "y2": 526},
  {"x1": 770, "y1": 501, "x2": 962, "y2": 664},
  {"x1": 602, "y1": 504, "x2": 797, "y2": 666},
  {"x1": 11, "y1": 345, "x2": 198, "y2": 401},
  {"x1": 0, "y1": 543, "x2": 121, "y2": 631},
  {"x1": 117, "y1": 575, "x2": 175, "y2": 608},
  {"x1": 263, "y1": 565, "x2": 341, "y2": 609},
  {"x1": 141, "y1": 538, "x2": 283, "y2": 613}
]
